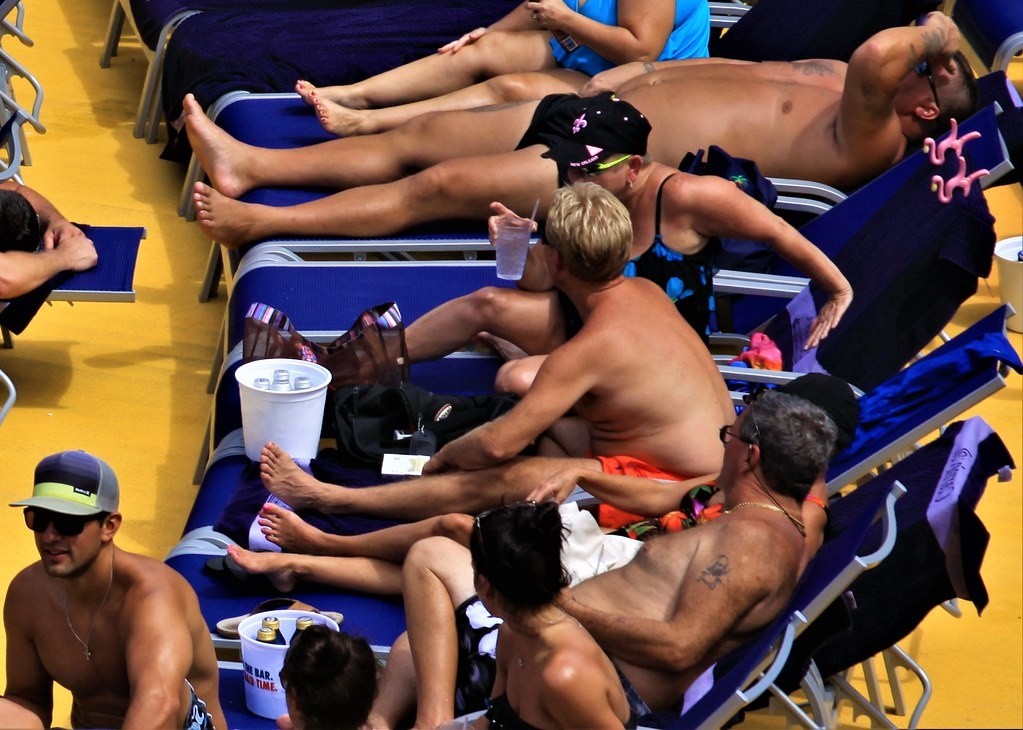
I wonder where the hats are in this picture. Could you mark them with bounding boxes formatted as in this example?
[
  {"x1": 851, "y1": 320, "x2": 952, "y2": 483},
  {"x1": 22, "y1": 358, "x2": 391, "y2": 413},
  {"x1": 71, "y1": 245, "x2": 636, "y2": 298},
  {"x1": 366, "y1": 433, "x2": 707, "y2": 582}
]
[
  {"x1": 540, "y1": 98, "x2": 652, "y2": 167},
  {"x1": 782, "y1": 373, "x2": 863, "y2": 440},
  {"x1": 8, "y1": 450, "x2": 120, "y2": 516}
]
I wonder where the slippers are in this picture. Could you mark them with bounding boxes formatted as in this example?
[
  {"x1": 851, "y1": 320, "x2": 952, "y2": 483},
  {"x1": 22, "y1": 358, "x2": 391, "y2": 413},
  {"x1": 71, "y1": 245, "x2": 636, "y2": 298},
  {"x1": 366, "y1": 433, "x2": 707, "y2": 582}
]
[
  {"x1": 217, "y1": 598, "x2": 344, "y2": 640},
  {"x1": 205, "y1": 552, "x2": 270, "y2": 580}
]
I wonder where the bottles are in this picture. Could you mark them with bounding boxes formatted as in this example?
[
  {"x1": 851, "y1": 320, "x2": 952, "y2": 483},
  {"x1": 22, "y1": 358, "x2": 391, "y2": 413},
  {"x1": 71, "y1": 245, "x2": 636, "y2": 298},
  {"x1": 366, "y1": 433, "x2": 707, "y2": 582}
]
[
  {"x1": 257, "y1": 615, "x2": 314, "y2": 645},
  {"x1": 254, "y1": 369, "x2": 312, "y2": 392},
  {"x1": 1017, "y1": 250, "x2": 1023, "y2": 262}
]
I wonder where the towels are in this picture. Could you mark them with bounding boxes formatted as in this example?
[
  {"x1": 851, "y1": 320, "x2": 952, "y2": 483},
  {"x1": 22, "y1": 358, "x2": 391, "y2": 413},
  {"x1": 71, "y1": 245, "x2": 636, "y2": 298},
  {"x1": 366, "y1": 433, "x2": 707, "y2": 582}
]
[{"x1": 556, "y1": 499, "x2": 645, "y2": 591}]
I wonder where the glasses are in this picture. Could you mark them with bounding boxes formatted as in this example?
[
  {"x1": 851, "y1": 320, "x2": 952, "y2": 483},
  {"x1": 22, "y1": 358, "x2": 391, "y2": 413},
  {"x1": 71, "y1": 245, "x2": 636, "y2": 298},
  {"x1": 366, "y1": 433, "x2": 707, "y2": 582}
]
[
  {"x1": 23, "y1": 506, "x2": 106, "y2": 536},
  {"x1": 719, "y1": 425, "x2": 750, "y2": 445},
  {"x1": 914, "y1": 59, "x2": 940, "y2": 108},
  {"x1": 579, "y1": 154, "x2": 632, "y2": 176},
  {"x1": 472, "y1": 499, "x2": 539, "y2": 554}
]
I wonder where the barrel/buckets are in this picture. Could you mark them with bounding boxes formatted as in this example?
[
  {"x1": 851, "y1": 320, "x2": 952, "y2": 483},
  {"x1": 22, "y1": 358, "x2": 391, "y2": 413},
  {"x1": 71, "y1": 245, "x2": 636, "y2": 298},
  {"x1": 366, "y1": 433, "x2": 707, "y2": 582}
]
[
  {"x1": 238, "y1": 610, "x2": 340, "y2": 721},
  {"x1": 993, "y1": 235, "x2": 1023, "y2": 335},
  {"x1": 234, "y1": 358, "x2": 333, "y2": 464}
]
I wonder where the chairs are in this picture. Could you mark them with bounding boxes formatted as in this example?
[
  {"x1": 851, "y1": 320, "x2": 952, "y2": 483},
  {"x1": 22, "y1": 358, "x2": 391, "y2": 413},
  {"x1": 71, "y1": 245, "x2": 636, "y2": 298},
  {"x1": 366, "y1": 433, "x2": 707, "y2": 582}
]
[{"x1": 0, "y1": 1, "x2": 1023, "y2": 730}]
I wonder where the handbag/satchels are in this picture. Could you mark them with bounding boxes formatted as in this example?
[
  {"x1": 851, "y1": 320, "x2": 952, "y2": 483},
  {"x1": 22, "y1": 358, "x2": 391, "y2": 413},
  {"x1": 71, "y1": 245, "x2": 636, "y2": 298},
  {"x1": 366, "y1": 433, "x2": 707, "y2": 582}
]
[{"x1": 333, "y1": 382, "x2": 522, "y2": 463}]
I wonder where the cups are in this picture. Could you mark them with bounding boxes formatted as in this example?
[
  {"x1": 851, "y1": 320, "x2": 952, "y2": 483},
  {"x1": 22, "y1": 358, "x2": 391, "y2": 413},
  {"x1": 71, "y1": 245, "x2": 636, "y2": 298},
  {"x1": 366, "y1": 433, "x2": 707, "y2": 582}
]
[{"x1": 495, "y1": 216, "x2": 534, "y2": 281}]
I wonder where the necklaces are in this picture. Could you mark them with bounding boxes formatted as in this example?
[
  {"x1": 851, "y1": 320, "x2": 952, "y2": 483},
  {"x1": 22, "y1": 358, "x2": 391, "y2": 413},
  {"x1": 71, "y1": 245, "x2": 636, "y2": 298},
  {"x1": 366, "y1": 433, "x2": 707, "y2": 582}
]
[
  {"x1": 724, "y1": 502, "x2": 785, "y2": 513},
  {"x1": 518, "y1": 656, "x2": 526, "y2": 667},
  {"x1": 64, "y1": 543, "x2": 113, "y2": 661}
]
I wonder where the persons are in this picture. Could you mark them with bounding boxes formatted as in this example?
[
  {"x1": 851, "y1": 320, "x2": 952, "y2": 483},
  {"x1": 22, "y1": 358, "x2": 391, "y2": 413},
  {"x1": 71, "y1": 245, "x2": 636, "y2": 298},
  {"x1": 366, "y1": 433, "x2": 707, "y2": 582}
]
[
  {"x1": 297, "y1": 0, "x2": 709, "y2": 135},
  {"x1": 181, "y1": 11, "x2": 979, "y2": 248},
  {"x1": 0, "y1": 180, "x2": 98, "y2": 300},
  {"x1": 276, "y1": 387, "x2": 836, "y2": 730},
  {"x1": 0, "y1": 449, "x2": 227, "y2": 730},
  {"x1": 228, "y1": 372, "x2": 859, "y2": 596},
  {"x1": 260, "y1": 180, "x2": 736, "y2": 529},
  {"x1": 279, "y1": 623, "x2": 390, "y2": 730},
  {"x1": 361, "y1": 96, "x2": 853, "y2": 398},
  {"x1": 437, "y1": 499, "x2": 637, "y2": 730}
]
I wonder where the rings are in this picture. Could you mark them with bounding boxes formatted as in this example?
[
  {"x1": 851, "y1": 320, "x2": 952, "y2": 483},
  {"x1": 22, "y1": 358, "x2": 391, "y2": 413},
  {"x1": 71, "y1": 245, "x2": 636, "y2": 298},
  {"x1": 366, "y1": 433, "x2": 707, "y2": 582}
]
[{"x1": 533, "y1": 14, "x2": 536, "y2": 20}]
